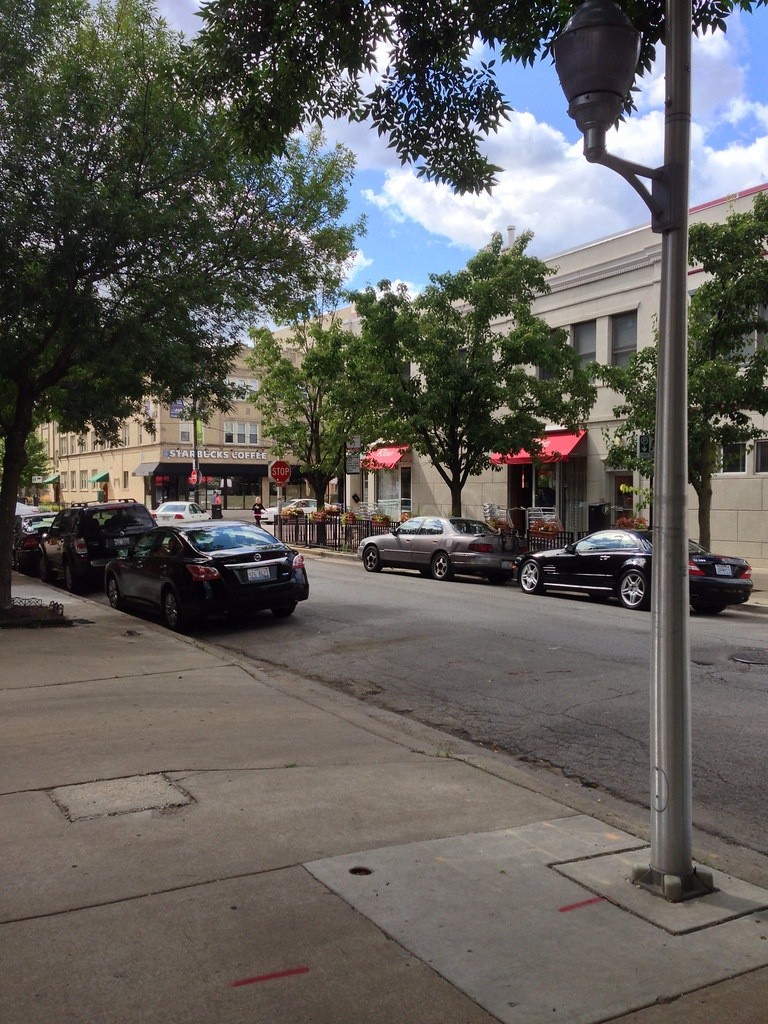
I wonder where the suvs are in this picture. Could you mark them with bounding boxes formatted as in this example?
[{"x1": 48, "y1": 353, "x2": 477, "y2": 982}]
[{"x1": 38, "y1": 498, "x2": 159, "y2": 596}]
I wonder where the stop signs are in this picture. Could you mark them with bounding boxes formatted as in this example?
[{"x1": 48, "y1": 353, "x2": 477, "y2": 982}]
[
  {"x1": 270, "y1": 461, "x2": 292, "y2": 484},
  {"x1": 191, "y1": 469, "x2": 198, "y2": 484}
]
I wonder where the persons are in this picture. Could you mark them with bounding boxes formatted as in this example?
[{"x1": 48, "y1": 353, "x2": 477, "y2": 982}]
[
  {"x1": 211, "y1": 490, "x2": 220, "y2": 504},
  {"x1": 252, "y1": 497, "x2": 267, "y2": 527}
]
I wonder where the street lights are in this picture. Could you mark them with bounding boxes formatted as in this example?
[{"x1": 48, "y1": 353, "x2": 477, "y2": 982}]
[{"x1": 551, "y1": 1, "x2": 717, "y2": 904}]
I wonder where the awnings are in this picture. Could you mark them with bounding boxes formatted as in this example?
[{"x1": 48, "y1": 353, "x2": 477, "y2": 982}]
[
  {"x1": 490, "y1": 430, "x2": 586, "y2": 463},
  {"x1": 43, "y1": 474, "x2": 60, "y2": 484},
  {"x1": 87, "y1": 472, "x2": 109, "y2": 482},
  {"x1": 359, "y1": 443, "x2": 409, "y2": 470},
  {"x1": 132, "y1": 462, "x2": 308, "y2": 477}
]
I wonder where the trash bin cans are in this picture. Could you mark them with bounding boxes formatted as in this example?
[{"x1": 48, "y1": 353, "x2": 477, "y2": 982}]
[{"x1": 212, "y1": 504, "x2": 221, "y2": 519}]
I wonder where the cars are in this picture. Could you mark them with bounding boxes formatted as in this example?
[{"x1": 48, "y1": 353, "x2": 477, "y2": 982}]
[
  {"x1": 260, "y1": 498, "x2": 341, "y2": 525},
  {"x1": 512, "y1": 527, "x2": 754, "y2": 615},
  {"x1": 104, "y1": 518, "x2": 310, "y2": 635},
  {"x1": 357, "y1": 515, "x2": 530, "y2": 586},
  {"x1": 149, "y1": 500, "x2": 212, "y2": 526},
  {"x1": 12, "y1": 511, "x2": 70, "y2": 577}
]
